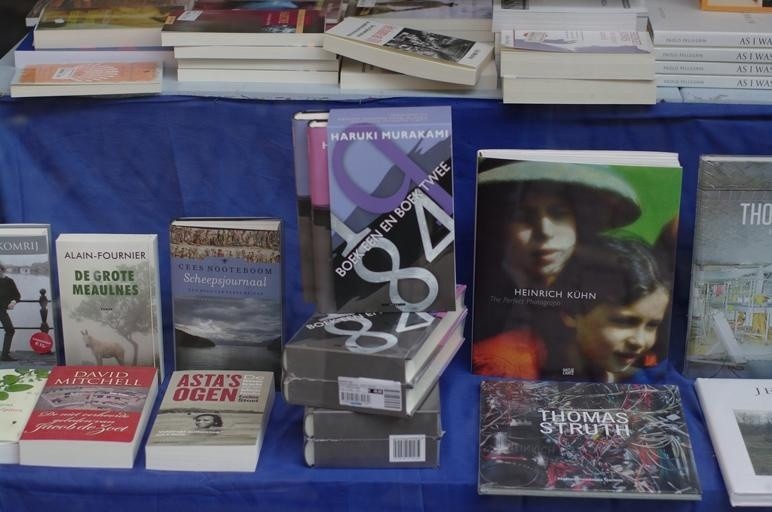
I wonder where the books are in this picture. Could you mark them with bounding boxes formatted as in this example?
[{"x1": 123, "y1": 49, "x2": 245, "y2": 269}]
[{"x1": 0, "y1": 0, "x2": 772, "y2": 507}]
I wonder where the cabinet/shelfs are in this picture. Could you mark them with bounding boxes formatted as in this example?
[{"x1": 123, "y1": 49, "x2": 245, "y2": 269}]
[{"x1": 0, "y1": 37, "x2": 771, "y2": 510}]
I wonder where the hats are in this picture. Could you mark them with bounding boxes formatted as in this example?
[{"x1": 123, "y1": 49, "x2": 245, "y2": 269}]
[{"x1": 478, "y1": 159, "x2": 639, "y2": 233}]
